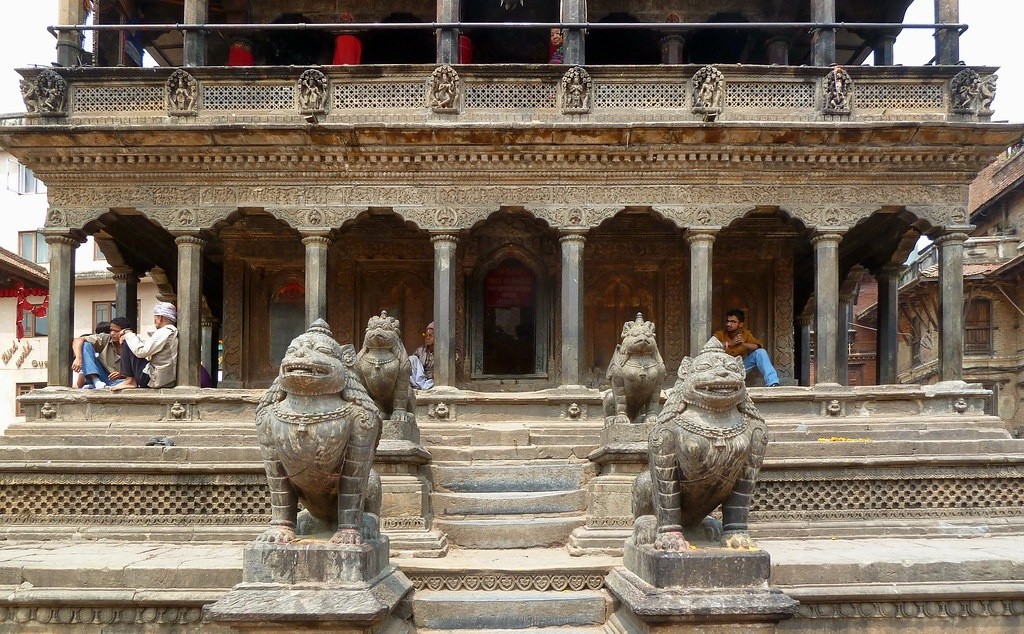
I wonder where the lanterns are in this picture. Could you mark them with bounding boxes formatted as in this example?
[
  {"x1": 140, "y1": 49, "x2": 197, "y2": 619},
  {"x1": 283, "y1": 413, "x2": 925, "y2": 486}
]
[
  {"x1": 228, "y1": 39, "x2": 255, "y2": 66},
  {"x1": 330, "y1": 34, "x2": 361, "y2": 65},
  {"x1": 458, "y1": 35, "x2": 471, "y2": 65},
  {"x1": 0, "y1": 278, "x2": 49, "y2": 339}
]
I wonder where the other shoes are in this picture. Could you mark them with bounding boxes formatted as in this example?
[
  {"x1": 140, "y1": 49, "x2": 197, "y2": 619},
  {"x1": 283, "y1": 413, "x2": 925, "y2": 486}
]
[{"x1": 771, "y1": 383, "x2": 779, "y2": 387}]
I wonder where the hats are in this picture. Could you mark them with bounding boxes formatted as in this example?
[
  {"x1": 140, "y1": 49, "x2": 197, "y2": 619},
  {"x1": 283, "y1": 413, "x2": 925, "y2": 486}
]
[
  {"x1": 153, "y1": 302, "x2": 177, "y2": 322},
  {"x1": 427, "y1": 321, "x2": 434, "y2": 330}
]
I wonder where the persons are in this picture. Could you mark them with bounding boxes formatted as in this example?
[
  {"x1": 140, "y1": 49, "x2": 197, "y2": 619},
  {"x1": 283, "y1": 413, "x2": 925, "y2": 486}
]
[
  {"x1": 569, "y1": 73, "x2": 583, "y2": 109},
  {"x1": 438, "y1": 73, "x2": 454, "y2": 108},
  {"x1": 409, "y1": 319, "x2": 464, "y2": 385},
  {"x1": 699, "y1": 76, "x2": 713, "y2": 107},
  {"x1": 176, "y1": 78, "x2": 189, "y2": 110},
  {"x1": 107, "y1": 301, "x2": 179, "y2": 391},
  {"x1": 712, "y1": 308, "x2": 780, "y2": 387},
  {"x1": 72, "y1": 316, "x2": 140, "y2": 389},
  {"x1": 40, "y1": 80, "x2": 58, "y2": 111},
  {"x1": 831, "y1": 71, "x2": 846, "y2": 110},
  {"x1": 960, "y1": 77, "x2": 972, "y2": 109},
  {"x1": 547, "y1": 28, "x2": 563, "y2": 64}
]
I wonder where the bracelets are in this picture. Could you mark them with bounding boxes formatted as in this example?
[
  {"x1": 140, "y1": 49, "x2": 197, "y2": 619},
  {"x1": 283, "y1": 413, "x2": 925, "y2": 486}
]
[{"x1": 725, "y1": 341, "x2": 729, "y2": 348}]
